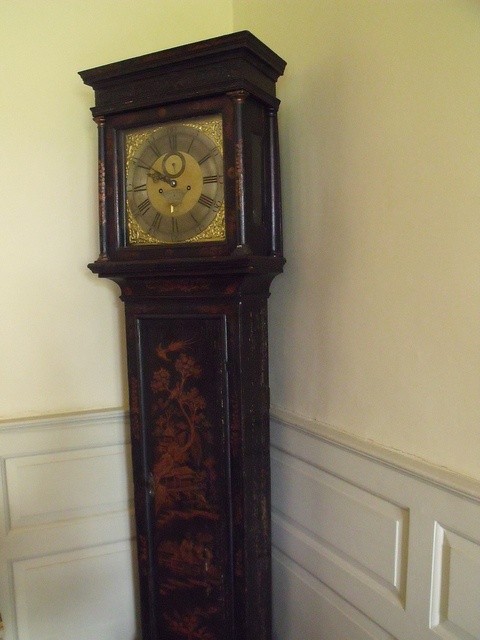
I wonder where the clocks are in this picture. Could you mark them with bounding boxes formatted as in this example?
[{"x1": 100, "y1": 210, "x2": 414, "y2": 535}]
[{"x1": 117, "y1": 111, "x2": 232, "y2": 251}]
